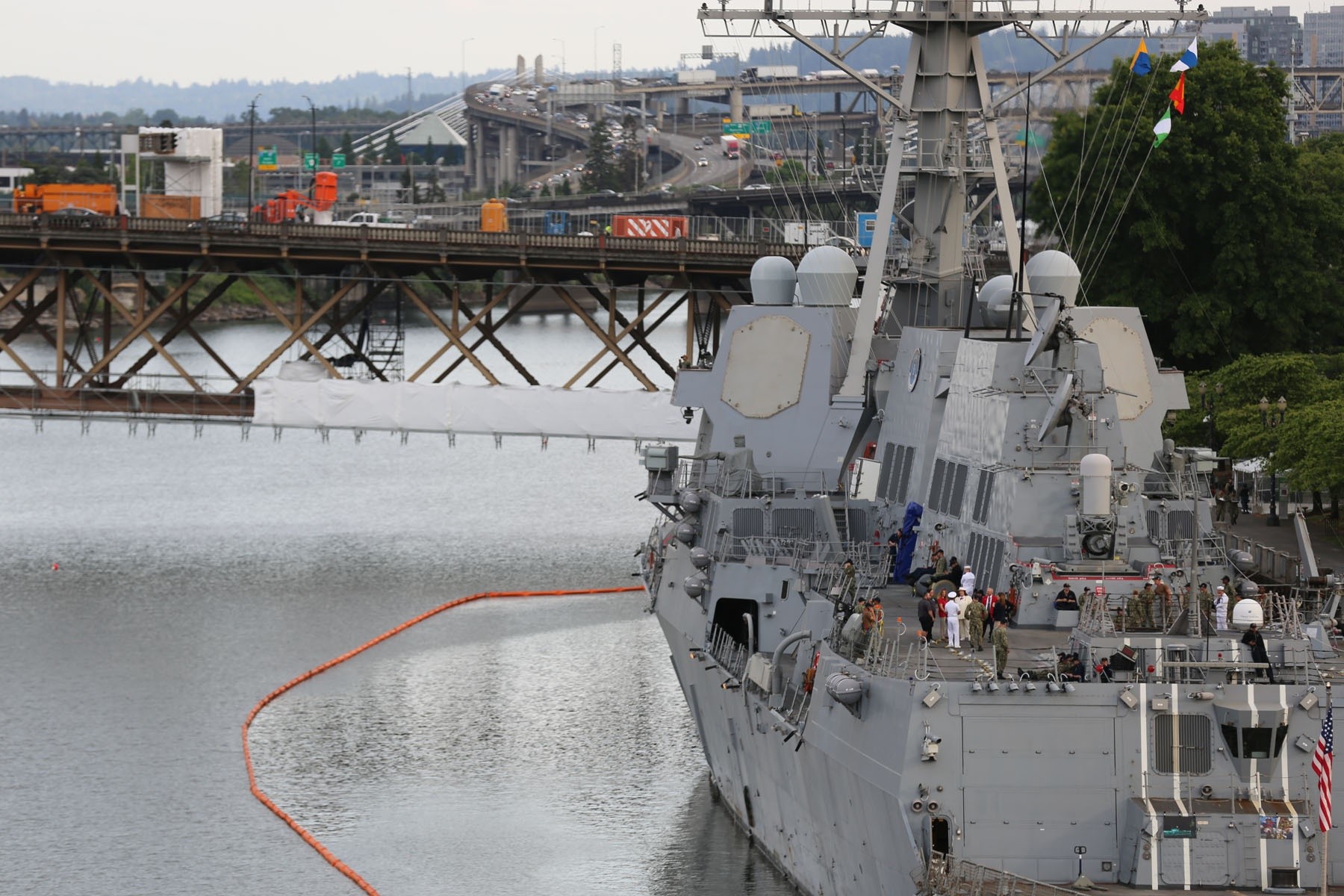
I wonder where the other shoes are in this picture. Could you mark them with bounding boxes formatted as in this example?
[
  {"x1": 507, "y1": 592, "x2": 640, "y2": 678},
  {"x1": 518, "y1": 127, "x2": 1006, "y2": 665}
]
[
  {"x1": 945, "y1": 645, "x2": 953, "y2": 648},
  {"x1": 997, "y1": 675, "x2": 1006, "y2": 679},
  {"x1": 1018, "y1": 667, "x2": 1027, "y2": 679},
  {"x1": 955, "y1": 646, "x2": 961, "y2": 648},
  {"x1": 979, "y1": 646, "x2": 983, "y2": 651},
  {"x1": 1231, "y1": 521, "x2": 1237, "y2": 526},
  {"x1": 1243, "y1": 511, "x2": 1249, "y2": 514}
]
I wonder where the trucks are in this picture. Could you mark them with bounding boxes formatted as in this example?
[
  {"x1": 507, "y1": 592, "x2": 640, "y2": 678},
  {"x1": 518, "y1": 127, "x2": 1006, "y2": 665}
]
[
  {"x1": 489, "y1": 84, "x2": 505, "y2": 100},
  {"x1": 720, "y1": 135, "x2": 740, "y2": 159},
  {"x1": 649, "y1": 65, "x2": 878, "y2": 88},
  {"x1": 12, "y1": 182, "x2": 120, "y2": 216}
]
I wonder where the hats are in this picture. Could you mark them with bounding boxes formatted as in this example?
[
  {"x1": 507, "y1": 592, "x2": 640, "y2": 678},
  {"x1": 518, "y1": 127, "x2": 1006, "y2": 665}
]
[
  {"x1": 858, "y1": 597, "x2": 867, "y2": 603},
  {"x1": 1153, "y1": 576, "x2": 1161, "y2": 581},
  {"x1": 964, "y1": 566, "x2": 971, "y2": 569},
  {"x1": 1250, "y1": 623, "x2": 1256, "y2": 627},
  {"x1": 1101, "y1": 658, "x2": 1108, "y2": 662},
  {"x1": 949, "y1": 561, "x2": 955, "y2": 567},
  {"x1": 1200, "y1": 583, "x2": 1207, "y2": 588},
  {"x1": 1063, "y1": 584, "x2": 1070, "y2": 587},
  {"x1": 1184, "y1": 583, "x2": 1191, "y2": 587},
  {"x1": 1217, "y1": 586, "x2": 1225, "y2": 591},
  {"x1": 1145, "y1": 583, "x2": 1152, "y2": 586},
  {"x1": 948, "y1": 592, "x2": 957, "y2": 598},
  {"x1": 1084, "y1": 587, "x2": 1090, "y2": 591},
  {"x1": 958, "y1": 587, "x2": 966, "y2": 591},
  {"x1": 1057, "y1": 652, "x2": 1078, "y2": 662},
  {"x1": 1221, "y1": 576, "x2": 1230, "y2": 581}
]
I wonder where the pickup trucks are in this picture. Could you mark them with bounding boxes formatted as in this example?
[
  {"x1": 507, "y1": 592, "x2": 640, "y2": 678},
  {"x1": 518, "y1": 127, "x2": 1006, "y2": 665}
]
[{"x1": 329, "y1": 213, "x2": 415, "y2": 230}]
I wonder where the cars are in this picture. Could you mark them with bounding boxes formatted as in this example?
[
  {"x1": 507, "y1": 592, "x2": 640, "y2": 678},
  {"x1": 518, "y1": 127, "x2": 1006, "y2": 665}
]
[
  {"x1": 692, "y1": 142, "x2": 704, "y2": 151},
  {"x1": 30, "y1": 207, "x2": 108, "y2": 228},
  {"x1": 696, "y1": 184, "x2": 727, "y2": 193},
  {"x1": 527, "y1": 163, "x2": 882, "y2": 256},
  {"x1": 744, "y1": 182, "x2": 773, "y2": 189},
  {"x1": 698, "y1": 157, "x2": 709, "y2": 165},
  {"x1": 475, "y1": 83, "x2": 632, "y2": 157},
  {"x1": 499, "y1": 196, "x2": 521, "y2": 203},
  {"x1": 188, "y1": 214, "x2": 251, "y2": 234}
]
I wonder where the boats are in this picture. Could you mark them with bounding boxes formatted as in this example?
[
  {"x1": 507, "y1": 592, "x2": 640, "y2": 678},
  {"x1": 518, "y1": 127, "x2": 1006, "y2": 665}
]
[{"x1": 633, "y1": 0, "x2": 1344, "y2": 894}]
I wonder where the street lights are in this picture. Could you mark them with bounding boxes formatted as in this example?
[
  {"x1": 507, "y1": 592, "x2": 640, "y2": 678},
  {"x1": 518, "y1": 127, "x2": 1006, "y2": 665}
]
[
  {"x1": 838, "y1": 114, "x2": 848, "y2": 194},
  {"x1": 302, "y1": 95, "x2": 317, "y2": 175},
  {"x1": 1256, "y1": 395, "x2": 1289, "y2": 526},
  {"x1": 552, "y1": 144, "x2": 563, "y2": 198},
  {"x1": 1197, "y1": 381, "x2": 1225, "y2": 496},
  {"x1": 526, "y1": 132, "x2": 542, "y2": 182},
  {"x1": 249, "y1": 93, "x2": 263, "y2": 211}
]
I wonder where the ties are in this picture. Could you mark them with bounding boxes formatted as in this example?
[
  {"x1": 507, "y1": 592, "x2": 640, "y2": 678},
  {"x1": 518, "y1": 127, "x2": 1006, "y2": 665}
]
[{"x1": 987, "y1": 596, "x2": 990, "y2": 610}]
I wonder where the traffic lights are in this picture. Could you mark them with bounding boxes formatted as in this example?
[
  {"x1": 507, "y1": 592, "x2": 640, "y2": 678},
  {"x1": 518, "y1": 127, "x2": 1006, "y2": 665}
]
[
  {"x1": 170, "y1": 133, "x2": 178, "y2": 153},
  {"x1": 155, "y1": 133, "x2": 169, "y2": 154}
]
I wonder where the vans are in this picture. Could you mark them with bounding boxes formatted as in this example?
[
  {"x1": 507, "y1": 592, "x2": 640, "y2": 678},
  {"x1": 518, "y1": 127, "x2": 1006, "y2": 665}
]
[
  {"x1": 0, "y1": 167, "x2": 36, "y2": 197},
  {"x1": 526, "y1": 91, "x2": 537, "y2": 102}
]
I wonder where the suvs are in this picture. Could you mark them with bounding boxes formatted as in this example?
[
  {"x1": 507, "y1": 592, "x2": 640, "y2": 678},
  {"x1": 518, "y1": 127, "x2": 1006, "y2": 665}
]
[{"x1": 702, "y1": 136, "x2": 715, "y2": 146}]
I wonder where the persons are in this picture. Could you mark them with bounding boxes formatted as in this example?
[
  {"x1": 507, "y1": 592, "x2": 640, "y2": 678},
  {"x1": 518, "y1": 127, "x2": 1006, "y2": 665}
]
[
  {"x1": 1152, "y1": 576, "x2": 1172, "y2": 628},
  {"x1": 1212, "y1": 586, "x2": 1229, "y2": 629},
  {"x1": 1138, "y1": 583, "x2": 1157, "y2": 627},
  {"x1": 1222, "y1": 575, "x2": 1237, "y2": 623},
  {"x1": 903, "y1": 549, "x2": 1015, "y2": 680},
  {"x1": 1216, "y1": 478, "x2": 1239, "y2": 526},
  {"x1": 843, "y1": 559, "x2": 857, "y2": 607},
  {"x1": 1113, "y1": 607, "x2": 1132, "y2": 629},
  {"x1": 888, "y1": 528, "x2": 902, "y2": 573},
  {"x1": 853, "y1": 597, "x2": 885, "y2": 663},
  {"x1": 1198, "y1": 582, "x2": 1212, "y2": 635},
  {"x1": 1241, "y1": 623, "x2": 1276, "y2": 682},
  {"x1": 1127, "y1": 590, "x2": 1144, "y2": 627},
  {"x1": 1239, "y1": 483, "x2": 1250, "y2": 514},
  {"x1": 1182, "y1": 582, "x2": 1190, "y2": 609},
  {"x1": 1079, "y1": 587, "x2": 1095, "y2": 620},
  {"x1": 1017, "y1": 652, "x2": 1112, "y2": 683},
  {"x1": 1054, "y1": 583, "x2": 1077, "y2": 611}
]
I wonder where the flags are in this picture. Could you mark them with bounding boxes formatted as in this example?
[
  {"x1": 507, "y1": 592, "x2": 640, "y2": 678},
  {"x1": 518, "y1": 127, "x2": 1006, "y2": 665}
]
[
  {"x1": 1129, "y1": 36, "x2": 1151, "y2": 77},
  {"x1": 1153, "y1": 104, "x2": 1171, "y2": 148},
  {"x1": 1168, "y1": 70, "x2": 1184, "y2": 115},
  {"x1": 1311, "y1": 698, "x2": 1333, "y2": 833},
  {"x1": 1169, "y1": 36, "x2": 1198, "y2": 72}
]
